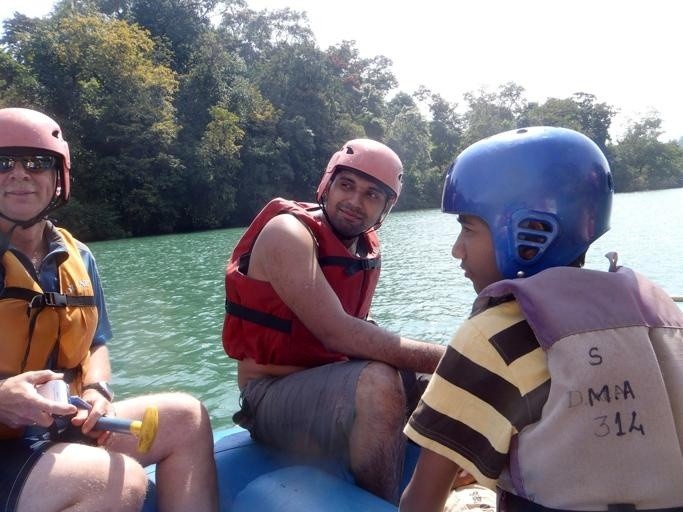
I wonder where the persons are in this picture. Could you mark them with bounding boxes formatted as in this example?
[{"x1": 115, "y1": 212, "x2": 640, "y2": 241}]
[
  {"x1": 0, "y1": 107, "x2": 221, "y2": 511},
  {"x1": 393, "y1": 125, "x2": 682, "y2": 510},
  {"x1": 222, "y1": 138, "x2": 477, "y2": 504}
]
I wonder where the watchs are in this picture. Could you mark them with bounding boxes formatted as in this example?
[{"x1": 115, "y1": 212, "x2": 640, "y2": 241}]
[{"x1": 77, "y1": 382, "x2": 116, "y2": 403}]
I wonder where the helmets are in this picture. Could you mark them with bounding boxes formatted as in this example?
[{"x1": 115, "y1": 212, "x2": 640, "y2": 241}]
[
  {"x1": 315, "y1": 137, "x2": 404, "y2": 227},
  {"x1": 440, "y1": 125, "x2": 615, "y2": 279},
  {"x1": 0, "y1": 107, "x2": 71, "y2": 202}
]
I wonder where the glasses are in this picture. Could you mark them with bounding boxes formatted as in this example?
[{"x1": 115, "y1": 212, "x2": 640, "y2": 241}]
[{"x1": 0, "y1": 156, "x2": 56, "y2": 174}]
